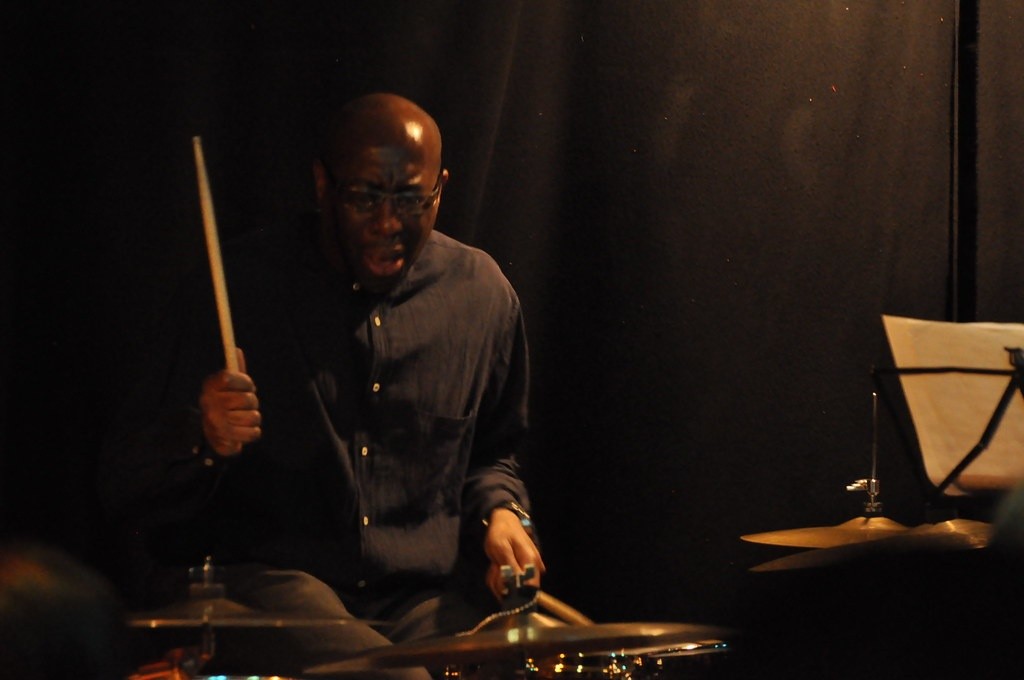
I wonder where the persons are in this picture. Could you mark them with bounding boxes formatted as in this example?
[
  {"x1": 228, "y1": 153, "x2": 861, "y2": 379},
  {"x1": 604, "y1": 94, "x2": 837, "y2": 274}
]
[{"x1": 197, "y1": 92, "x2": 546, "y2": 680}]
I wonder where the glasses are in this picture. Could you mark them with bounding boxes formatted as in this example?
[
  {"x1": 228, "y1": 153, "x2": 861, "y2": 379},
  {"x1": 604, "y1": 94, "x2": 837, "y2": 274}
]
[{"x1": 324, "y1": 161, "x2": 443, "y2": 215}]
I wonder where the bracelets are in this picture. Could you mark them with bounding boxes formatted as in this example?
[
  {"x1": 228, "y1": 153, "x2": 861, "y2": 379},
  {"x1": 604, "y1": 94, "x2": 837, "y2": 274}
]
[{"x1": 506, "y1": 501, "x2": 536, "y2": 537}]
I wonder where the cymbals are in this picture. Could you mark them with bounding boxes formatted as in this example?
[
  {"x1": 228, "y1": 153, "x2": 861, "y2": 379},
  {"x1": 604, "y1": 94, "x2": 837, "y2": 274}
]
[
  {"x1": 747, "y1": 516, "x2": 996, "y2": 573},
  {"x1": 119, "y1": 595, "x2": 350, "y2": 632},
  {"x1": 298, "y1": 620, "x2": 738, "y2": 675},
  {"x1": 738, "y1": 514, "x2": 910, "y2": 549}
]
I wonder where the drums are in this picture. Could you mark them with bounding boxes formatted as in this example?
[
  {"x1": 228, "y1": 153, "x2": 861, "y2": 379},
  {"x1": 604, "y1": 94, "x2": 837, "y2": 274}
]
[{"x1": 525, "y1": 642, "x2": 738, "y2": 680}]
